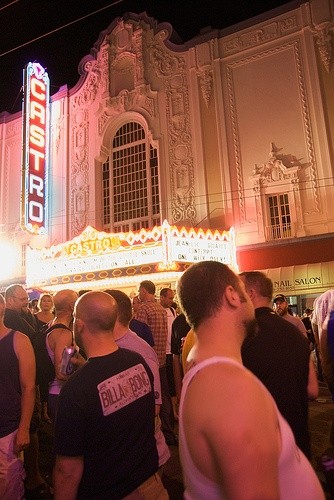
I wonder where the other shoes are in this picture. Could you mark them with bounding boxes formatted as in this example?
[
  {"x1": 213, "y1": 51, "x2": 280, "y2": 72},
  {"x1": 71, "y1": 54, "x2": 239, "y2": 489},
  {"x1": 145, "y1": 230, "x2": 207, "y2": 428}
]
[
  {"x1": 42, "y1": 416, "x2": 52, "y2": 424},
  {"x1": 164, "y1": 433, "x2": 175, "y2": 445}
]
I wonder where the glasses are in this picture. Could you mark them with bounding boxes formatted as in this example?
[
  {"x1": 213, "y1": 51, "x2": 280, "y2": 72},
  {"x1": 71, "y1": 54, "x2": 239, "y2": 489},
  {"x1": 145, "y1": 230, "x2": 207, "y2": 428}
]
[{"x1": 9, "y1": 295, "x2": 28, "y2": 302}]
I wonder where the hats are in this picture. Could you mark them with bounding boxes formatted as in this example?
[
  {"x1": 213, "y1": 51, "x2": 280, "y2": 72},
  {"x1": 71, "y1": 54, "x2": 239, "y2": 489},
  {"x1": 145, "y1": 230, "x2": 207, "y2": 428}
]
[{"x1": 273, "y1": 295, "x2": 286, "y2": 303}]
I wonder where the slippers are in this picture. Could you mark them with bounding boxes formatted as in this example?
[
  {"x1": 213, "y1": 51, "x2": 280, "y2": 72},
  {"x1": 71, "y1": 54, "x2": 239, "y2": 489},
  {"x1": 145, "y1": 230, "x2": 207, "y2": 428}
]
[{"x1": 25, "y1": 480, "x2": 55, "y2": 498}]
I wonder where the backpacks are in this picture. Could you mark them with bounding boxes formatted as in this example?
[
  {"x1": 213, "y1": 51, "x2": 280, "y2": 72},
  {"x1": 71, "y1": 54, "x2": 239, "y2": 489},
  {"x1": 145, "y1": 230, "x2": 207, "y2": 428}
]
[{"x1": 31, "y1": 323, "x2": 73, "y2": 386}]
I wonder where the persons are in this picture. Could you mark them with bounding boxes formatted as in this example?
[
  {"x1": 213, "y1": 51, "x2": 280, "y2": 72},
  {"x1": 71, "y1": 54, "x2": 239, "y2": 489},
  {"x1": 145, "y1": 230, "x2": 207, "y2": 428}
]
[{"x1": 0, "y1": 261, "x2": 334, "y2": 500}]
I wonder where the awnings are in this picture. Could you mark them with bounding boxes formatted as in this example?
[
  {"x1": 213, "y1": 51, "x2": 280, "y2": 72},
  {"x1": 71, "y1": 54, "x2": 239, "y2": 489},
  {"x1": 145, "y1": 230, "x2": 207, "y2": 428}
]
[{"x1": 249, "y1": 262, "x2": 334, "y2": 297}]
